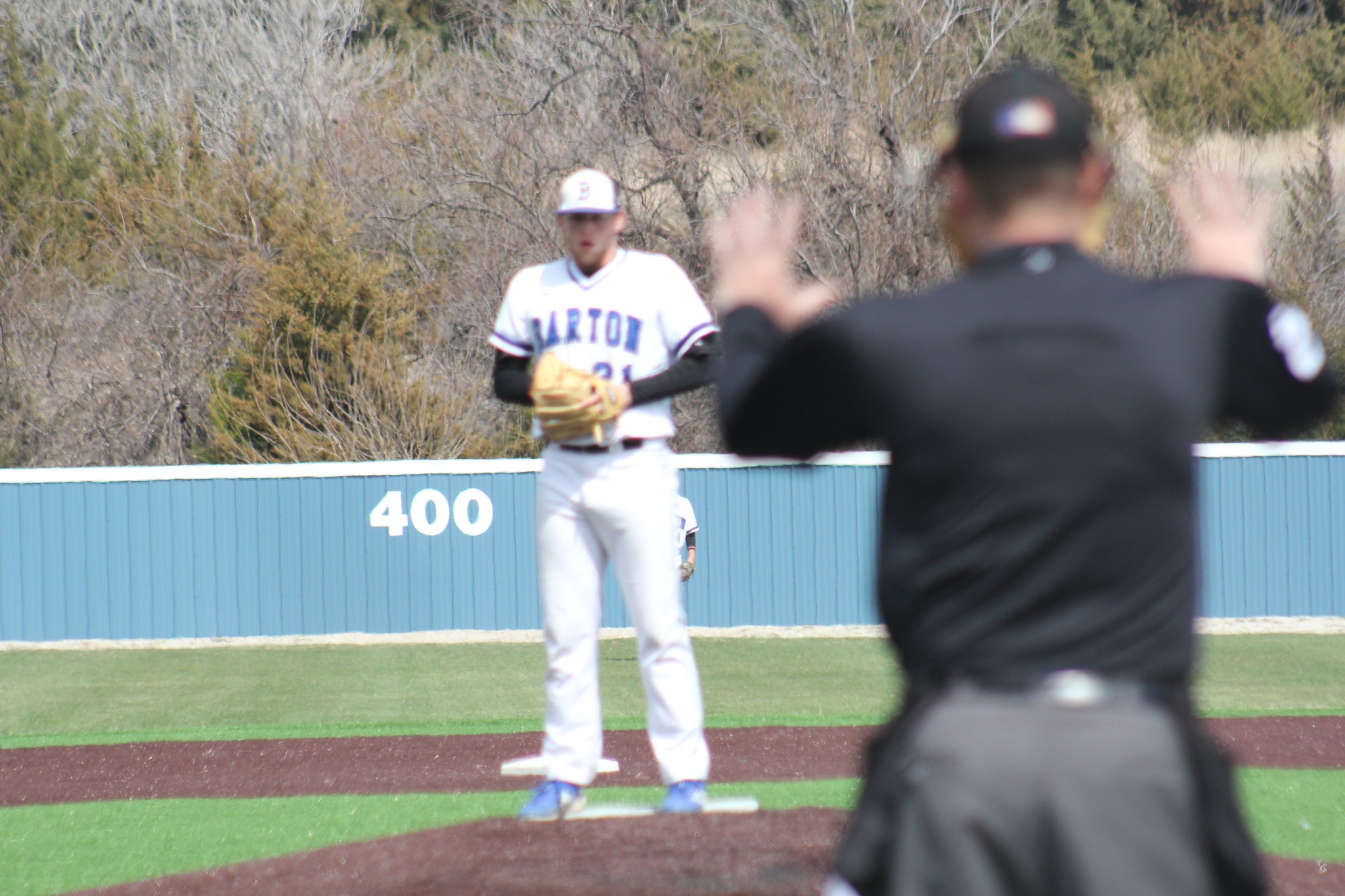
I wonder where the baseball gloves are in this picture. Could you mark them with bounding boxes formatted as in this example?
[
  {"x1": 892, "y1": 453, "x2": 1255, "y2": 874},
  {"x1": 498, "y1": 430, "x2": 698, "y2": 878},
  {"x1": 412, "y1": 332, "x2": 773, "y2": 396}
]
[{"x1": 528, "y1": 349, "x2": 624, "y2": 443}]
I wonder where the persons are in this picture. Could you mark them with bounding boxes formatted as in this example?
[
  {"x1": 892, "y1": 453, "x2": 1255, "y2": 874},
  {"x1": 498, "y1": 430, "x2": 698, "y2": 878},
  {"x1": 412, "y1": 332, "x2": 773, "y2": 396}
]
[
  {"x1": 677, "y1": 495, "x2": 699, "y2": 581},
  {"x1": 488, "y1": 169, "x2": 720, "y2": 823},
  {"x1": 708, "y1": 64, "x2": 1345, "y2": 896}
]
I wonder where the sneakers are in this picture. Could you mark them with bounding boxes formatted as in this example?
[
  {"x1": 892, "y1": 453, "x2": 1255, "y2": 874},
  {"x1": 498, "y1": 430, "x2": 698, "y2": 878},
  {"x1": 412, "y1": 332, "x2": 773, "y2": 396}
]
[
  {"x1": 655, "y1": 781, "x2": 708, "y2": 814},
  {"x1": 514, "y1": 781, "x2": 585, "y2": 822}
]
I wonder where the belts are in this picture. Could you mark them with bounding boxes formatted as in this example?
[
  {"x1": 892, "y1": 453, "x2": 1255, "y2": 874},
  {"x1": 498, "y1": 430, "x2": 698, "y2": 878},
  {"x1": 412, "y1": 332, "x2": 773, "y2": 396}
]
[
  {"x1": 561, "y1": 439, "x2": 642, "y2": 453},
  {"x1": 973, "y1": 665, "x2": 1165, "y2": 707}
]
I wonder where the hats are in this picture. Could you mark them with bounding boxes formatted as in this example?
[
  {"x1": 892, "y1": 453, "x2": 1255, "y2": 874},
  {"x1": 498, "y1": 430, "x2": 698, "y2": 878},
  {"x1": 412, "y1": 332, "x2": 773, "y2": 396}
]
[
  {"x1": 933, "y1": 67, "x2": 1091, "y2": 167},
  {"x1": 553, "y1": 168, "x2": 618, "y2": 214}
]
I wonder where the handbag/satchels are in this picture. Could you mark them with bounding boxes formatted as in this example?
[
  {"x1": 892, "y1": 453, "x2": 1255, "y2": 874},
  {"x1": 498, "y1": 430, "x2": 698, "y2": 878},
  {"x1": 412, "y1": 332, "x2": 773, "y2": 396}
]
[
  {"x1": 1162, "y1": 694, "x2": 1277, "y2": 895},
  {"x1": 818, "y1": 696, "x2": 926, "y2": 896}
]
